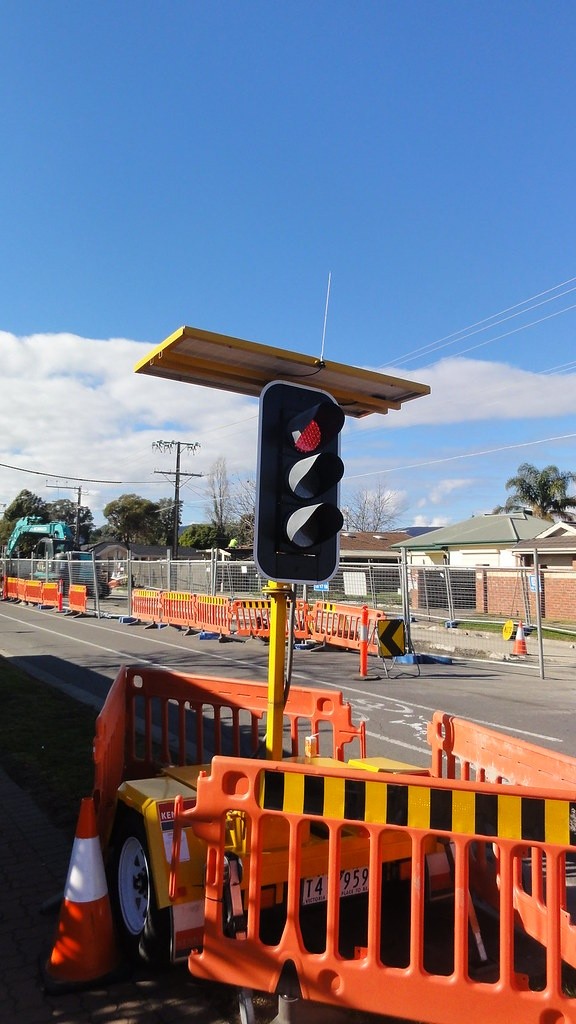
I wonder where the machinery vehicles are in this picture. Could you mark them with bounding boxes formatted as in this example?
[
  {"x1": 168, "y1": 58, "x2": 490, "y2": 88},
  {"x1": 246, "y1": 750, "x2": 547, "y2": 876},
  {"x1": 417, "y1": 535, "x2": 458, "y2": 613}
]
[{"x1": 0, "y1": 515, "x2": 111, "y2": 598}]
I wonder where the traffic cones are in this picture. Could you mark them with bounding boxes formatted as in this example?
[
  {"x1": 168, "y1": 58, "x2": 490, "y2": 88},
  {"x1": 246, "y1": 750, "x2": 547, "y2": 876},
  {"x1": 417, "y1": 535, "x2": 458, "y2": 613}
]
[
  {"x1": 36, "y1": 795, "x2": 135, "y2": 997},
  {"x1": 510, "y1": 619, "x2": 531, "y2": 657}
]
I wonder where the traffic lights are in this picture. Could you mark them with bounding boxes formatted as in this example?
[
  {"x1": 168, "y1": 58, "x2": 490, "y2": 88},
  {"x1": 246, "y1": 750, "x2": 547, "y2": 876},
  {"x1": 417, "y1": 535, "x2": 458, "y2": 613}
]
[{"x1": 252, "y1": 379, "x2": 345, "y2": 585}]
